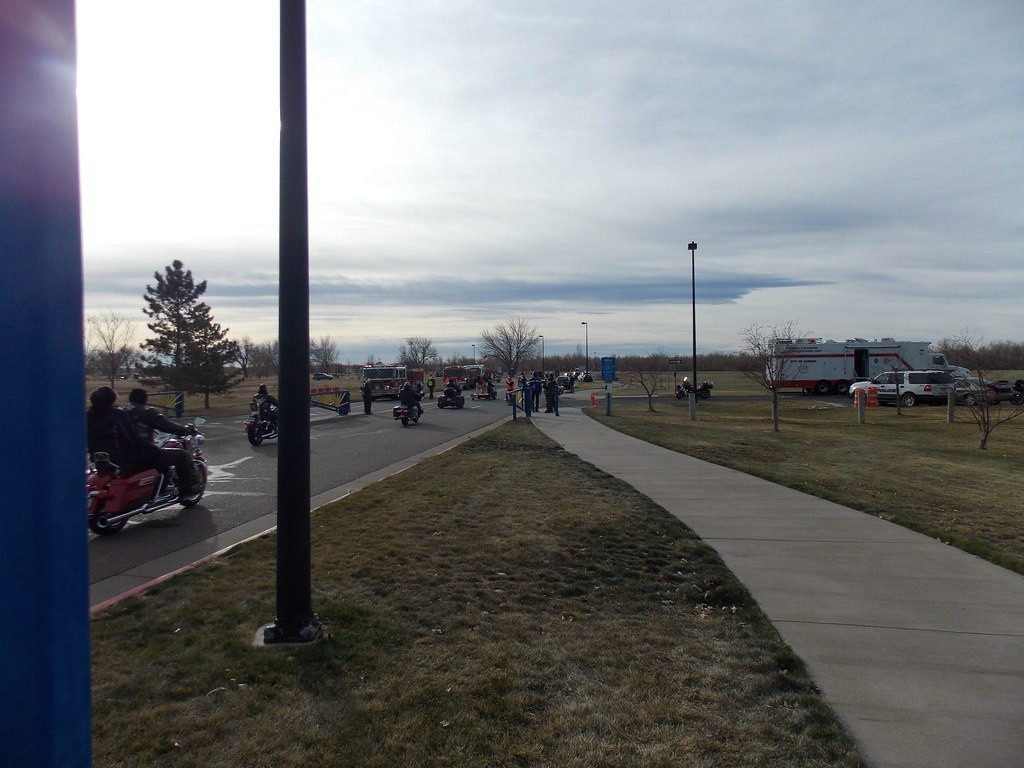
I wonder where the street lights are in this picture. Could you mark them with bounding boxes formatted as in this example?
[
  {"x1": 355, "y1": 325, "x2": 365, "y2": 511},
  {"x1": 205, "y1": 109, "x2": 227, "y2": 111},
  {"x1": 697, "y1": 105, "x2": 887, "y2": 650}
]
[
  {"x1": 582, "y1": 322, "x2": 588, "y2": 381},
  {"x1": 472, "y1": 344, "x2": 475, "y2": 365},
  {"x1": 688, "y1": 241, "x2": 698, "y2": 403},
  {"x1": 539, "y1": 335, "x2": 545, "y2": 379}
]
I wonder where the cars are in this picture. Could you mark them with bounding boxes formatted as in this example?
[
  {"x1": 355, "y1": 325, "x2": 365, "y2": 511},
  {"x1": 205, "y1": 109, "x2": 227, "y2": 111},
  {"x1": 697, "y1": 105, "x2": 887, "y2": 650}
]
[
  {"x1": 582, "y1": 374, "x2": 593, "y2": 382},
  {"x1": 555, "y1": 381, "x2": 564, "y2": 395},
  {"x1": 953, "y1": 377, "x2": 1024, "y2": 406}
]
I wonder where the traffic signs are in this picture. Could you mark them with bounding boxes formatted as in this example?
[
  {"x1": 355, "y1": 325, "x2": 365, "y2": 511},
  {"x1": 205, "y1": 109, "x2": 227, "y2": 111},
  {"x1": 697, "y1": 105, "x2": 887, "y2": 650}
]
[{"x1": 669, "y1": 360, "x2": 683, "y2": 364}]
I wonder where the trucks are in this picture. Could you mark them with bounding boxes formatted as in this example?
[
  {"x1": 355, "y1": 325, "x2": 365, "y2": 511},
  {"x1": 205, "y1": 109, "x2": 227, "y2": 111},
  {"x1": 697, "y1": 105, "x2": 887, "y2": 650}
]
[
  {"x1": 362, "y1": 363, "x2": 424, "y2": 401},
  {"x1": 443, "y1": 366, "x2": 478, "y2": 389},
  {"x1": 466, "y1": 365, "x2": 485, "y2": 379}
]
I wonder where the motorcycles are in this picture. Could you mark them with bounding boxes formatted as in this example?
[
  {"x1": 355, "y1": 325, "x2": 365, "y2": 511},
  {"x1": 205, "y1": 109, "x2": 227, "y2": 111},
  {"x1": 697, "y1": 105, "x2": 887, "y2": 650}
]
[
  {"x1": 393, "y1": 390, "x2": 425, "y2": 425},
  {"x1": 471, "y1": 381, "x2": 497, "y2": 401},
  {"x1": 676, "y1": 376, "x2": 713, "y2": 399},
  {"x1": 242, "y1": 404, "x2": 279, "y2": 446},
  {"x1": 86, "y1": 417, "x2": 207, "y2": 535},
  {"x1": 437, "y1": 387, "x2": 465, "y2": 409}
]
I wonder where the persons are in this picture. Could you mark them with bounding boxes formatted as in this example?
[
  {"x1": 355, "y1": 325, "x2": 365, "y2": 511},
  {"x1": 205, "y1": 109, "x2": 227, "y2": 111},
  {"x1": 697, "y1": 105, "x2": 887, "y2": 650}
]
[
  {"x1": 253, "y1": 384, "x2": 279, "y2": 426},
  {"x1": 86, "y1": 387, "x2": 171, "y2": 488},
  {"x1": 445, "y1": 378, "x2": 459, "y2": 389},
  {"x1": 427, "y1": 375, "x2": 435, "y2": 399},
  {"x1": 505, "y1": 372, "x2": 557, "y2": 413},
  {"x1": 468, "y1": 374, "x2": 496, "y2": 400},
  {"x1": 362, "y1": 383, "x2": 372, "y2": 415},
  {"x1": 124, "y1": 389, "x2": 202, "y2": 497},
  {"x1": 399, "y1": 382, "x2": 424, "y2": 415}
]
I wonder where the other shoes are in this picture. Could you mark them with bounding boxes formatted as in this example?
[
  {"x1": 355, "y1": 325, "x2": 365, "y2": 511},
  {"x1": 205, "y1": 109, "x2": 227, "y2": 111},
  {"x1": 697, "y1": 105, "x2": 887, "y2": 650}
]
[
  {"x1": 187, "y1": 484, "x2": 204, "y2": 496},
  {"x1": 165, "y1": 487, "x2": 173, "y2": 493}
]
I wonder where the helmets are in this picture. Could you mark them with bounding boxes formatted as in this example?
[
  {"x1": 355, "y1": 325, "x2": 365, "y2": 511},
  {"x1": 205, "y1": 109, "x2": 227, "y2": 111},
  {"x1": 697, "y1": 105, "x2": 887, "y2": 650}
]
[
  {"x1": 404, "y1": 382, "x2": 411, "y2": 386},
  {"x1": 90, "y1": 386, "x2": 117, "y2": 406},
  {"x1": 129, "y1": 389, "x2": 147, "y2": 401},
  {"x1": 260, "y1": 384, "x2": 267, "y2": 392}
]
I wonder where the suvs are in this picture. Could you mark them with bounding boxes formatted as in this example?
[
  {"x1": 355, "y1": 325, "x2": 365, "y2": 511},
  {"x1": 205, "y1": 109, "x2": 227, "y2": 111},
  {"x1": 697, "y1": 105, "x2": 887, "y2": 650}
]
[
  {"x1": 849, "y1": 369, "x2": 957, "y2": 407},
  {"x1": 313, "y1": 373, "x2": 333, "y2": 380},
  {"x1": 531, "y1": 371, "x2": 543, "y2": 379},
  {"x1": 555, "y1": 376, "x2": 570, "y2": 387},
  {"x1": 573, "y1": 368, "x2": 591, "y2": 380}
]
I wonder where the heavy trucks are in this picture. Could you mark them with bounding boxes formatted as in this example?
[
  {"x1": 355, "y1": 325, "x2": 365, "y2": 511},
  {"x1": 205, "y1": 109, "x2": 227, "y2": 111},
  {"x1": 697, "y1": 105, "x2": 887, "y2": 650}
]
[{"x1": 766, "y1": 338, "x2": 971, "y2": 396}]
